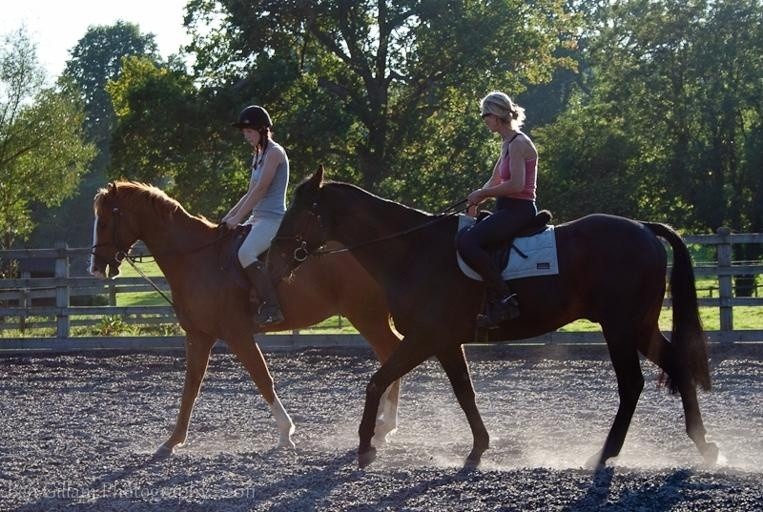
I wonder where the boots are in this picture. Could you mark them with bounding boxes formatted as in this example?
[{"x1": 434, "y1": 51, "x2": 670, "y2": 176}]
[{"x1": 243, "y1": 261, "x2": 285, "y2": 325}]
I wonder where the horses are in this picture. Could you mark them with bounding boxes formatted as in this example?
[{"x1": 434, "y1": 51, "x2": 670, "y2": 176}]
[
  {"x1": 264, "y1": 162, "x2": 721, "y2": 472},
  {"x1": 88, "y1": 176, "x2": 405, "y2": 455}
]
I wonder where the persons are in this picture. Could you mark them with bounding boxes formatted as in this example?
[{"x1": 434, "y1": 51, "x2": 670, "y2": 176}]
[
  {"x1": 222, "y1": 104, "x2": 290, "y2": 335},
  {"x1": 459, "y1": 90, "x2": 543, "y2": 323}
]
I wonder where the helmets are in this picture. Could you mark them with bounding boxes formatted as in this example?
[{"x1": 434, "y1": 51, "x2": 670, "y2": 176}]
[{"x1": 232, "y1": 106, "x2": 272, "y2": 128}]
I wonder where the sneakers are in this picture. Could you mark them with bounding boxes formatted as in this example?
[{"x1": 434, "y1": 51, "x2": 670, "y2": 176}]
[{"x1": 479, "y1": 298, "x2": 521, "y2": 325}]
